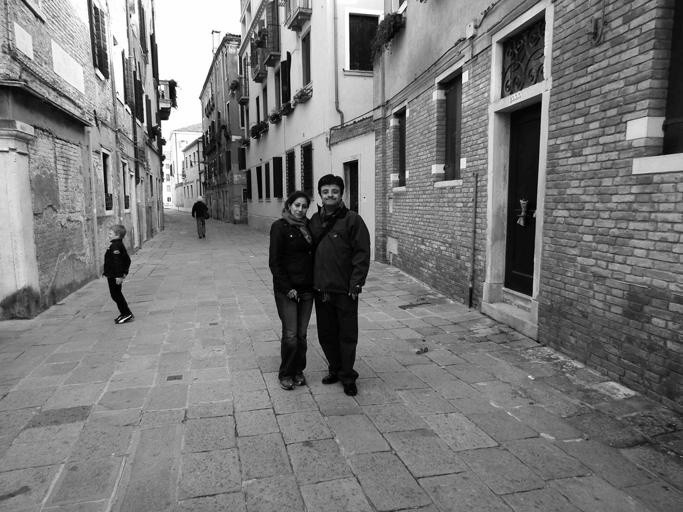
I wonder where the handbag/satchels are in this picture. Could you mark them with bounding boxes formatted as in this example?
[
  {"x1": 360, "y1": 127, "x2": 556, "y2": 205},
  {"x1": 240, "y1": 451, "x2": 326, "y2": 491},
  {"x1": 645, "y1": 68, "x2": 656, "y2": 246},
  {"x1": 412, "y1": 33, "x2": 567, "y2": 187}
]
[{"x1": 205, "y1": 212, "x2": 209, "y2": 219}]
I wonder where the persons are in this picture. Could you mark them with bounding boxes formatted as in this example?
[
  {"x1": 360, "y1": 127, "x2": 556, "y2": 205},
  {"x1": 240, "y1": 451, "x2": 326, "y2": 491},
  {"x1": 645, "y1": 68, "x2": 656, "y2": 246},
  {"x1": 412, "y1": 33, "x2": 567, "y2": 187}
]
[
  {"x1": 266, "y1": 191, "x2": 313, "y2": 391},
  {"x1": 190, "y1": 196, "x2": 208, "y2": 239},
  {"x1": 307, "y1": 173, "x2": 371, "y2": 396},
  {"x1": 101, "y1": 225, "x2": 135, "y2": 324}
]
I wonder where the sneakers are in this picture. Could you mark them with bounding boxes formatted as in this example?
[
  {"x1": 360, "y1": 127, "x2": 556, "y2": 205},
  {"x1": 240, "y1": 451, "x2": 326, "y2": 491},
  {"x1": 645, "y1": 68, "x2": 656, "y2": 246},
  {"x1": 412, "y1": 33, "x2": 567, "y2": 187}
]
[
  {"x1": 278, "y1": 370, "x2": 305, "y2": 390},
  {"x1": 322, "y1": 372, "x2": 339, "y2": 384},
  {"x1": 199, "y1": 234, "x2": 205, "y2": 239},
  {"x1": 342, "y1": 378, "x2": 357, "y2": 395},
  {"x1": 115, "y1": 312, "x2": 135, "y2": 324}
]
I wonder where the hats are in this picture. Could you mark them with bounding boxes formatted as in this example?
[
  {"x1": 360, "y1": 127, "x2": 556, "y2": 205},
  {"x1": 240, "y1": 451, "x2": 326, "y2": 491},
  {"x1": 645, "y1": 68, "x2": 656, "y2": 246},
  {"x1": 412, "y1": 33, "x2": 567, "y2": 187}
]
[{"x1": 197, "y1": 196, "x2": 203, "y2": 201}]
